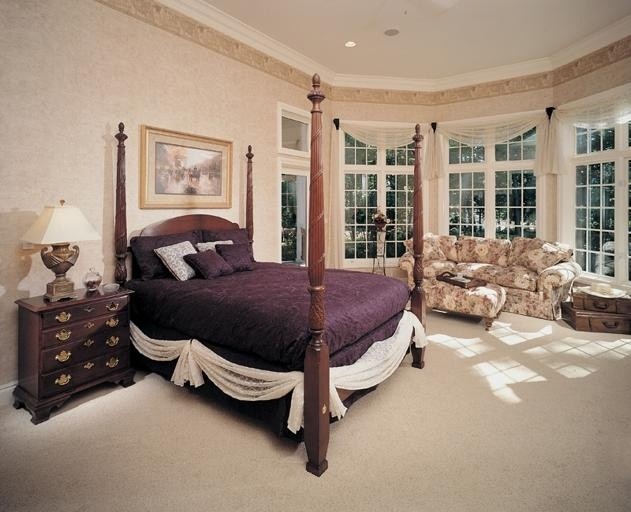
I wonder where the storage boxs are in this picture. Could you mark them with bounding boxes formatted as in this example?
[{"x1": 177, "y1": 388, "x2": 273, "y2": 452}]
[{"x1": 561, "y1": 291, "x2": 631, "y2": 334}]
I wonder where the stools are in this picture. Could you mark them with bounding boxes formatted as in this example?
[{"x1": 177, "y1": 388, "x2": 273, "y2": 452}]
[{"x1": 422, "y1": 276, "x2": 507, "y2": 331}]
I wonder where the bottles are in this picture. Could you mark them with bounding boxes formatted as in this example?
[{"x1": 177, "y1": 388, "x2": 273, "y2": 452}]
[{"x1": 83, "y1": 267, "x2": 102, "y2": 292}]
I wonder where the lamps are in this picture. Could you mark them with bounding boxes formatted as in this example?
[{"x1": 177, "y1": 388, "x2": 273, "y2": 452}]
[{"x1": 20, "y1": 199, "x2": 103, "y2": 303}]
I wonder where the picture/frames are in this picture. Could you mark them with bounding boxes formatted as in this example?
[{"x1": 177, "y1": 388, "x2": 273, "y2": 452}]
[{"x1": 139, "y1": 123, "x2": 235, "y2": 210}]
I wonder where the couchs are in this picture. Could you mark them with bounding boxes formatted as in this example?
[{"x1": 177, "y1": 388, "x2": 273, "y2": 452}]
[{"x1": 397, "y1": 235, "x2": 583, "y2": 331}]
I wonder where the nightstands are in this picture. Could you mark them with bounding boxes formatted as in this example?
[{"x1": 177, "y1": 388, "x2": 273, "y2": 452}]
[{"x1": 12, "y1": 283, "x2": 136, "y2": 425}]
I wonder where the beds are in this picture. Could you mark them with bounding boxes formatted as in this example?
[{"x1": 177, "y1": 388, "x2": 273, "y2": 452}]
[{"x1": 125, "y1": 214, "x2": 412, "y2": 442}]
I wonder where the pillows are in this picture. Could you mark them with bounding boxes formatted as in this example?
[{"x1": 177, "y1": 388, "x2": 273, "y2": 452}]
[
  {"x1": 404, "y1": 232, "x2": 570, "y2": 275},
  {"x1": 130, "y1": 228, "x2": 256, "y2": 283}
]
